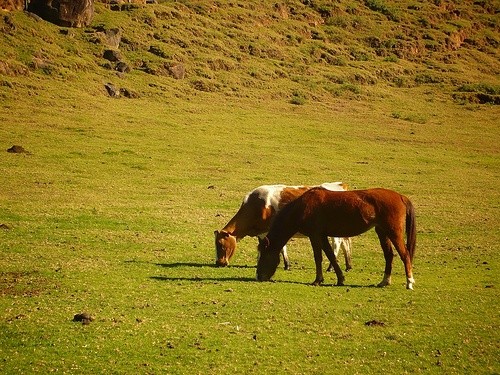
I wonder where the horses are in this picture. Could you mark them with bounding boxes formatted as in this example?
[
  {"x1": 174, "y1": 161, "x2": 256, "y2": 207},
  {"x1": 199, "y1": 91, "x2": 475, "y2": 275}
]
[
  {"x1": 254, "y1": 186, "x2": 417, "y2": 289},
  {"x1": 213, "y1": 181, "x2": 352, "y2": 271}
]
